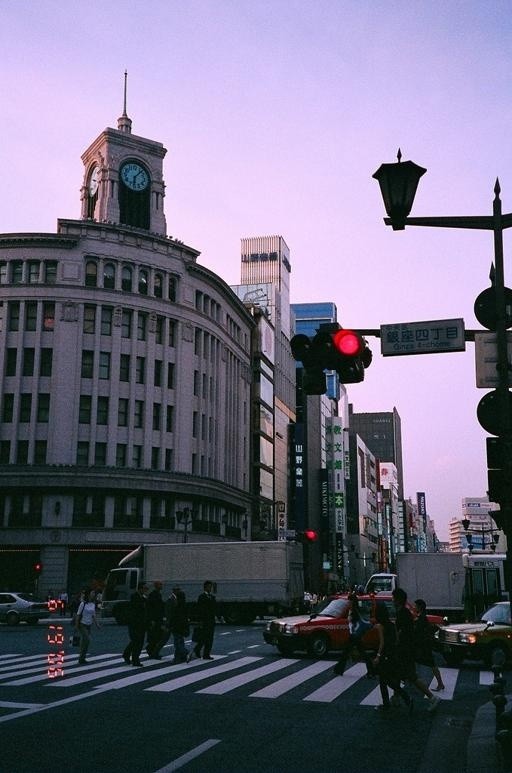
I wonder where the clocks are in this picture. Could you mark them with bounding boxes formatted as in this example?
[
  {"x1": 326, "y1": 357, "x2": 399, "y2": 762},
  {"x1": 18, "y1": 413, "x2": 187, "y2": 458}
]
[
  {"x1": 86, "y1": 165, "x2": 102, "y2": 198},
  {"x1": 119, "y1": 160, "x2": 151, "y2": 193}
]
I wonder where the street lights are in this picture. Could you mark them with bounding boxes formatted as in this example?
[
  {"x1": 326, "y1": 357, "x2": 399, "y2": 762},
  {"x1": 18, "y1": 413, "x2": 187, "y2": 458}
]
[
  {"x1": 175, "y1": 506, "x2": 200, "y2": 543},
  {"x1": 355, "y1": 551, "x2": 377, "y2": 581},
  {"x1": 461, "y1": 516, "x2": 504, "y2": 552},
  {"x1": 370, "y1": 148, "x2": 511, "y2": 773}
]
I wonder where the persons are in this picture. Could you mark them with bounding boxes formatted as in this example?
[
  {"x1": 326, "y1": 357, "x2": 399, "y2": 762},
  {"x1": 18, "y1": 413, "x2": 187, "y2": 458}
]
[
  {"x1": 333, "y1": 595, "x2": 376, "y2": 679},
  {"x1": 413, "y1": 599, "x2": 445, "y2": 690},
  {"x1": 122, "y1": 580, "x2": 192, "y2": 666},
  {"x1": 302, "y1": 591, "x2": 328, "y2": 613},
  {"x1": 373, "y1": 606, "x2": 413, "y2": 711},
  {"x1": 193, "y1": 580, "x2": 221, "y2": 659},
  {"x1": 390, "y1": 589, "x2": 441, "y2": 712},
  {"x1": 47, "y1": 588, "x2": 102, "y2": 626},
  {"x1": 336, "y1": 584, "x2": 367, "y2": 595},
  {"x1": 74, "y1": 589, "x2": 101, "y2": 664}
]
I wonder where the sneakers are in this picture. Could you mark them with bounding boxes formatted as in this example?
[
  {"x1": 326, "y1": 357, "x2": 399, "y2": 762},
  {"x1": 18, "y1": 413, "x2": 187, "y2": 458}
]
[
  {"x1": 427, "y1": 696, "x2": 440, "y2": 710},
  {"x1": 404, "y1": 695, "x2": 414, "y2": 713}
]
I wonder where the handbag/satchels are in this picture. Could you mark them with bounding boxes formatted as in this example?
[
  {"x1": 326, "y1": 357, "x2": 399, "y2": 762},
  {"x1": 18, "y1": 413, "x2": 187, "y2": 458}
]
[
  {"x1": 70, "y1": 600, "x2": 86, "y2": 628},
  {"x1": 352, "y1": 607, "x2": 373, "y2": 641}
]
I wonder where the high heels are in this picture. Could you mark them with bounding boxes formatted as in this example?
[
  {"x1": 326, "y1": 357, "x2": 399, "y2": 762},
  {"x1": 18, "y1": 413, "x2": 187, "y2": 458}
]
[{"x1": 431, "y1": 684, "x2": 445, "y2": 692}]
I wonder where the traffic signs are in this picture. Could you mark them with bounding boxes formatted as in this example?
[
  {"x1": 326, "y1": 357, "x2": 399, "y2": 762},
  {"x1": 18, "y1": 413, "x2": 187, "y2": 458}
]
[{"x1": 378, "y1": 313, "x2": 467, "y2": 357}]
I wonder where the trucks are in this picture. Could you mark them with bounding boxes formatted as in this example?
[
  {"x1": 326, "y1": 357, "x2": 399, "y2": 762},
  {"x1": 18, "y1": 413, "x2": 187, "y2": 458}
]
[
  {"x1": 100, "y1": 540, "x2": 305, "y2": 626},
  {"x1": 363, "y1": 552, "x2": 511, "y2": 623}
]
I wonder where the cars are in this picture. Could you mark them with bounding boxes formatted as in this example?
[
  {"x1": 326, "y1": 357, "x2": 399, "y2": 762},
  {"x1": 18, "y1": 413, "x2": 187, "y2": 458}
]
[
  {"x1": 0, "y1": 592, "x2": 50, "y2": 627},
  {"x1": 431, "y1": 601, "x2": 512, "y2": 671},
  {"x1": 263, "y1": 594, "x2": 448, "y2": 660}
]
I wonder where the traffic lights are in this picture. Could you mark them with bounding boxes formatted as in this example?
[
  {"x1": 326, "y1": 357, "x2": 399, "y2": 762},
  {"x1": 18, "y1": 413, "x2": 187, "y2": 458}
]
[
  {"x1": 35, "y1": 563, "x2": 42, "y2": 576},
  {"x1": 290, "y1": 326, "x2": 363, "y2": 362},
  {"x1": 305, "y1": 529, "x2": 321, "y2": 543}
]
